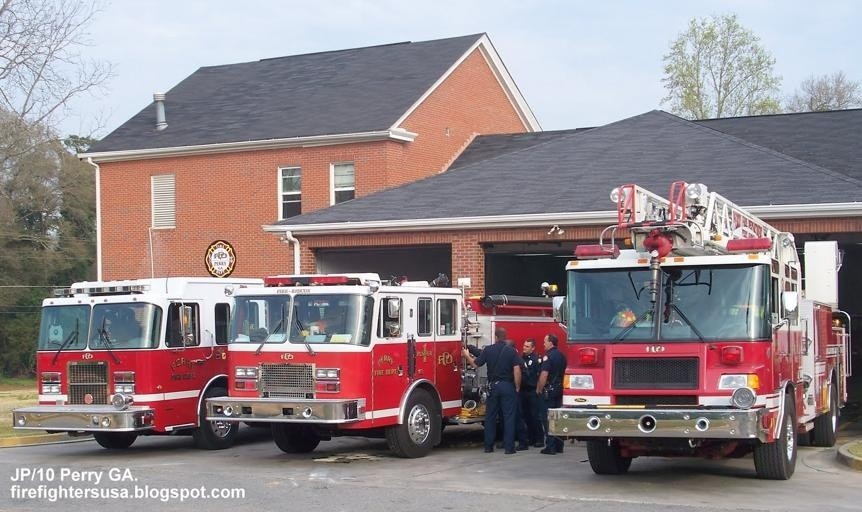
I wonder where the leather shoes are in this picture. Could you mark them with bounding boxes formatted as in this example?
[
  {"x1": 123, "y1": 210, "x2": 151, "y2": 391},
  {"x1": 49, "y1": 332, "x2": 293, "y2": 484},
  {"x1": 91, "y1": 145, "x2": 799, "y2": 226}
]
[{"x1": 485, "y1": 442, "x2": 563, "y2": 454}]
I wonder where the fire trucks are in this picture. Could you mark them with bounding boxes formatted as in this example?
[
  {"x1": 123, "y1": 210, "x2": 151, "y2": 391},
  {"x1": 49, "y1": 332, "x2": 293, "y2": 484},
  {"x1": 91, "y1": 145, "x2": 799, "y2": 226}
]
[
  {"x1": 203, "y1": 272, "x2": 568, "y2": 461},
  {"x1": 548, "y1": 178, "x2": 855, "y2": 483},
  {"x1": 11, "y1": 276, "x2": 266, "y2": 450}
]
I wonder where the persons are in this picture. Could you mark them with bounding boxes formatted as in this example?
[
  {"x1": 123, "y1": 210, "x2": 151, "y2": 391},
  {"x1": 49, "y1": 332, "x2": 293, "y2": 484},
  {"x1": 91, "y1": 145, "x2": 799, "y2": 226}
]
[
  {"x1": 521, "y1": 338, "x2": 545, "y2": 447},
  {"x1": 467, "y1": 339, "x2": 529, "y2": 451},
  {"x1": 536, "y1": 334, "x2": 567, "y2": 455},
  {"x1": 461, "y1": 327, "x2": 521, "y2": 454}
]
